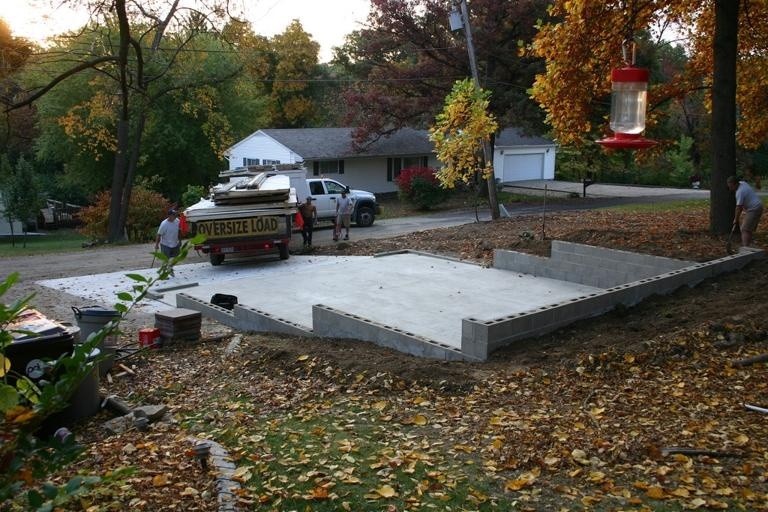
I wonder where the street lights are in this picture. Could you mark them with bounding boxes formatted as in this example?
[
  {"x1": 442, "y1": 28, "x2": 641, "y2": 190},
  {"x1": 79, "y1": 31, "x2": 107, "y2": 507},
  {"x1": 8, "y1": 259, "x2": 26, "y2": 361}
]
[{"x1": 448, "y1": 0, "x2": 499, "y2": 219}]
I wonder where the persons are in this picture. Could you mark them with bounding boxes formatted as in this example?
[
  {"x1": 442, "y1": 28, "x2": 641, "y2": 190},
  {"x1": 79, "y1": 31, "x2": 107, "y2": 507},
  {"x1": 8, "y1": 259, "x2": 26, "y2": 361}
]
[
  {"x1": 726, "y1": 176, "x2": 764, "y2": 247},
  {"x1": 155, "y1": 208, "x2": 181, "y2": 280},
  {"x1": 298, "y1": 196, "x2": 317, "y2": 247},
  {"x1": 332, "y1": 191, "x2": 353, "y2": 241}
]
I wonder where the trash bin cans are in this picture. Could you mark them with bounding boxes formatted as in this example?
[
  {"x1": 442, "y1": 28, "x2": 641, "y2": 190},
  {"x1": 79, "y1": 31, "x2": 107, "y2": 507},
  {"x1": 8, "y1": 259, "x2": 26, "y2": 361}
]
[{"x1": 0, "y1": 305, "x2": 121, "y2": 441}]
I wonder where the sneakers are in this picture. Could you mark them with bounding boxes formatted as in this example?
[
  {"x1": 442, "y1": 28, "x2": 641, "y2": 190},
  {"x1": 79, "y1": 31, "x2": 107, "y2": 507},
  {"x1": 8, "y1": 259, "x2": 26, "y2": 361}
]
[{"x1": 332, "y1": 234, "x2": 350, "y2": 241}]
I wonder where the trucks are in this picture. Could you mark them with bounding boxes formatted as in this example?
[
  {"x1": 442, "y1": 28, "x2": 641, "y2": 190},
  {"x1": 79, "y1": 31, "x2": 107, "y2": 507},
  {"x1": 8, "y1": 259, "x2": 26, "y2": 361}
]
[{"x1": 183, "y1": 169, "x2": 291, "y2": 265}]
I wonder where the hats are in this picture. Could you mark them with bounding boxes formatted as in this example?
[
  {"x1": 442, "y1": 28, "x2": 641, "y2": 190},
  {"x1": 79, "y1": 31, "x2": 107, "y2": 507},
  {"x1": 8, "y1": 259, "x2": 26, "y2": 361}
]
[
  {"x1": 168, "y1": 209, "x2": 177, "y2": 215},
  {"x1": 726, "y1": 175, "x2": 742, "y2": 184}
]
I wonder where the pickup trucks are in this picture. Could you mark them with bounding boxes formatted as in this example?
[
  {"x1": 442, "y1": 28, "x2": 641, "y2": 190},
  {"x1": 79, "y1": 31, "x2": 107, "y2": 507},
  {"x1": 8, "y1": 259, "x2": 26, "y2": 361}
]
[{"x1": 219, "y1": 167, "x2": 384, "y2": 227}]
